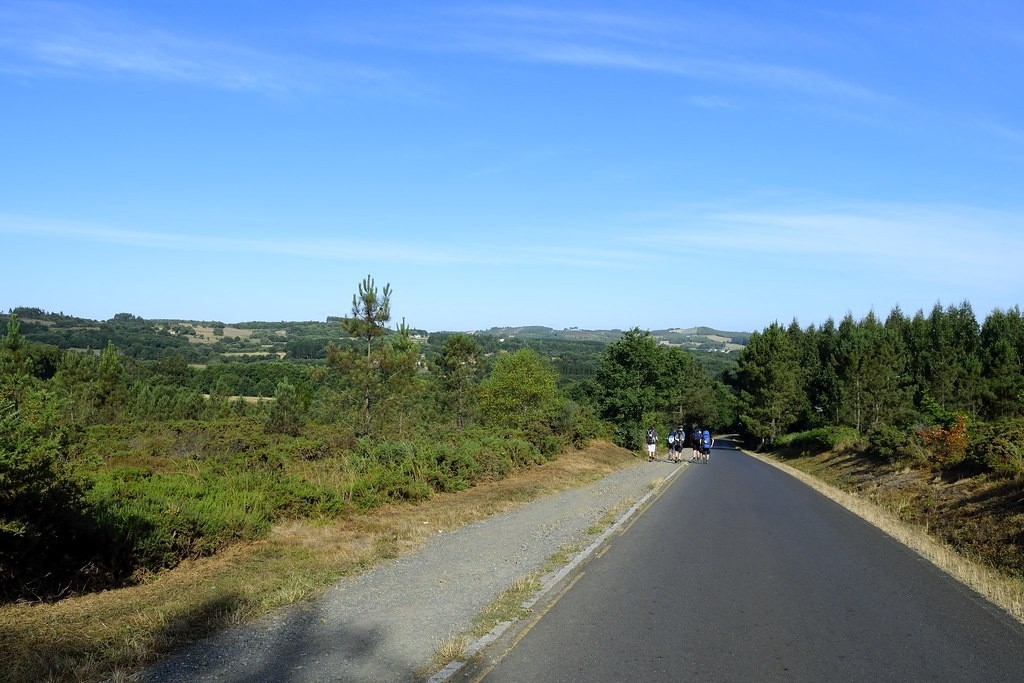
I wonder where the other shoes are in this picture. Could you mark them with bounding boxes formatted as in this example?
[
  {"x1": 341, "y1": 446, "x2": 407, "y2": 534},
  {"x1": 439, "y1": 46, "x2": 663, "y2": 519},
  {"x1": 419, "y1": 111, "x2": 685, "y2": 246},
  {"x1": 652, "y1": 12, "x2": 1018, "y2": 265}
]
[
  {"x1": 675, "y1": 457, "x2": 677, "y2": 463},
  {"x1": 649, "y1": 456, "x2": 652, "y2": 462},
  {"x1": 693, "y1": 457, "x2": 696, "y2": 459}
]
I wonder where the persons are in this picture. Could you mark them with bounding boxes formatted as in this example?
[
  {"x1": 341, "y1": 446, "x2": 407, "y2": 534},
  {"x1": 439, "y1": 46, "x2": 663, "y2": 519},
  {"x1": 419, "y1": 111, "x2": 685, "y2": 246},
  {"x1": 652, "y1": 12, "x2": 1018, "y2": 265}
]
[
  {"x1": 646, "y1": 427, "x2": 658, "y2": 462},
  {"x1": 689, "y1": 427, "x2": 714, "y2": 464},
  {"x1": 666, "y1": 425, "x2": 685, "y2": 464}
]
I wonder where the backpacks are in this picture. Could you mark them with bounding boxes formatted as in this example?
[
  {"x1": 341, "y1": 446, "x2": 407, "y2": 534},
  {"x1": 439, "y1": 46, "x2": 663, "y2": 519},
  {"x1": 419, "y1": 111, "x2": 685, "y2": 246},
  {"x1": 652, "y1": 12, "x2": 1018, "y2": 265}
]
[
  {"x1": 693, "y1": 430, "x2": 700, "y2": 440},
  {"x1": 701, "y1": 430, "x2": 710, "y2": 448},
  {"x1": 646, "y1": 428, "x2": 655, "y2": 444},
  {"x1": 668, "y1": 435, "x2": 674, "y2": 443}
]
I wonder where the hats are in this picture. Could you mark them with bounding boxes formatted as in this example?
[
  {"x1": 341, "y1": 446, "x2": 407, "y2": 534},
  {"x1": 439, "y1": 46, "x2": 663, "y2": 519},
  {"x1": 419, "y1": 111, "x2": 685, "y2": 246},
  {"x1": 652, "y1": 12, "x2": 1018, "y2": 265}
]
[{"x1": 678, "y1": 425, "x2": 683, "y2": 429}]
